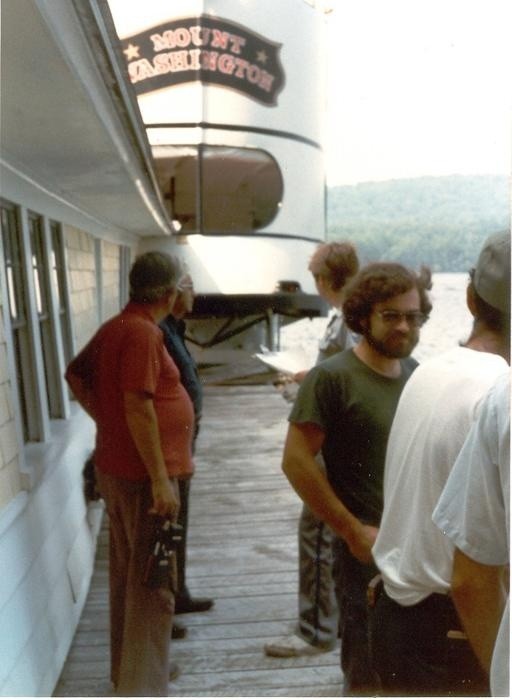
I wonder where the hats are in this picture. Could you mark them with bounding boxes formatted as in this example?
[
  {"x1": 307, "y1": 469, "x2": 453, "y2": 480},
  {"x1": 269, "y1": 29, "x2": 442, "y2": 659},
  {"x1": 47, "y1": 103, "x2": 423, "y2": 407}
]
[{"x1": 473, "y1": 229, "x2": 511, "y2": 316}]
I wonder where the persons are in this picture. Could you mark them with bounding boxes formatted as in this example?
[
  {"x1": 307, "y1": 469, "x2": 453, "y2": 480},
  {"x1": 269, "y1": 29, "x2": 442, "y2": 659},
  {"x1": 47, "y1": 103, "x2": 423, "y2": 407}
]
[
  {"x1": 432, "y1": 373, "x2": 510, "y2": 698},
  {"x1": 258, "y1": 238, "x2": 374, "y2": 657},
  {"x1": 153, "y1": 258, "x2": 214, "y2": 641},
  {"x1": 370, "y1": 231, "x2": 509, "y2": 698},
  {"x1": 62, "y1": 247, "x2": 197, "y2": 698},
  {"x1": 277, "y1": 259, "x2": 437, "y2": 696}
]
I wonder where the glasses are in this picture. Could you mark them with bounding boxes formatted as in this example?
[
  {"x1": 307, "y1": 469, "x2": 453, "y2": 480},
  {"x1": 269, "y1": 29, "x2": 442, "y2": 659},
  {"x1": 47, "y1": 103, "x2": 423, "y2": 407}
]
[{"x1": 371, "y1": 311, "x2": 426, "y2": 326}]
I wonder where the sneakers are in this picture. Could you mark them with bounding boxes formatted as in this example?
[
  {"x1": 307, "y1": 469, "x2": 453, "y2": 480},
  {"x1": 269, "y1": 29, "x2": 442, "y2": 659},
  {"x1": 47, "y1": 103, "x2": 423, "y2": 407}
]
[
  {"x1": 264, "y1": 632, "x2": 336, "y2": 657},
  {"x1": 168, "y1": 623, "x2": 186, "y2": 681},
  {"x1": 173, "y1": 597, "x2": 214, "y2": 615}
]
